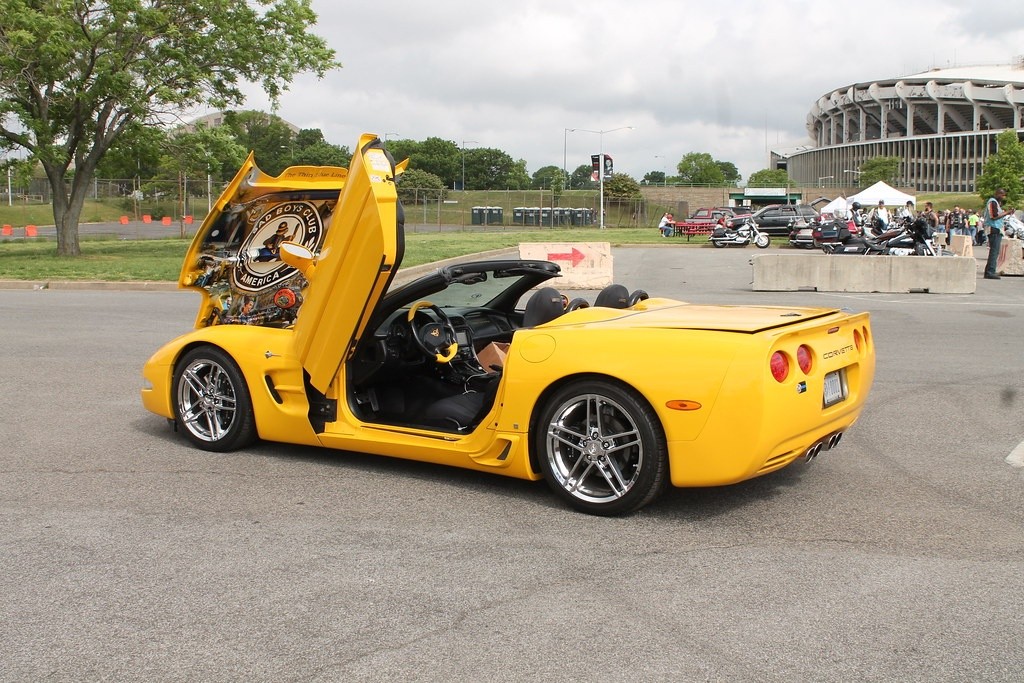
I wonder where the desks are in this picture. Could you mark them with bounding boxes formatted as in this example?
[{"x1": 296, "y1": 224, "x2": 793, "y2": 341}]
[{"x1": 677, "y1": 222, "x2": 716, "y2": 236}]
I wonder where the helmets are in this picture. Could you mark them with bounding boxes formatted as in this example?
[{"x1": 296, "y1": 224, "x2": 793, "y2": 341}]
[{"x1": 852, "y1": 202, "x2": 862, "y2": 211}]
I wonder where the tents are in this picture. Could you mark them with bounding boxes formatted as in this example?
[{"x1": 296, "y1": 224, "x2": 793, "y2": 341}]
[
  {"x1": 846, "y1": 180, "x2": 916, "y2": 210},
  {"x1": 821, "y1": 197, "x2": 846, "y2": 215}
]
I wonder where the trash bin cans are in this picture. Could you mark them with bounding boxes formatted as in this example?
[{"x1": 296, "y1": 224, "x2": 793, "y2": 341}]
[
  {"x1": 513, "y1": 207, "x2": 591, "y2": 227},
  {"x1": 471, "y1": 206, "x2": 503, "y2": 224}
]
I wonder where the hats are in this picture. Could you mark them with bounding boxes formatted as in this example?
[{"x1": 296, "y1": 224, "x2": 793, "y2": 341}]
[{"x1": 879, "y1": 200, "x2": 884, "y2": 204}]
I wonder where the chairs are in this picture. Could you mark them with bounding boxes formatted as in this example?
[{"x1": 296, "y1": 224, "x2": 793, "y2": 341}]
[
  {"x1": 425, "y1": 286, "x2": 564, "y2": 427},
  {"x1": 592, "y1": 284, "x2": 628, "y2": 309}
]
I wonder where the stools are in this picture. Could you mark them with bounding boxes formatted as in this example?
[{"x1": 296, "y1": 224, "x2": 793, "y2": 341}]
[{"x1": 659, "y1": 228, "x2": 664, "y2": 236}]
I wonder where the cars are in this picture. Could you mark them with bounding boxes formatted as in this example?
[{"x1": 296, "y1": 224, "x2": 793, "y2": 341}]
[
  {"x1": 732, "y1": 204, "x2": 821, "y2": 236},
  {"x1": 693, "y1": 208, "x2": 752, "y2": 219}
]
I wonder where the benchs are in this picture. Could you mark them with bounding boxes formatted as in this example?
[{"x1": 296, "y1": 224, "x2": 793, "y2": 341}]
[{"x1": 674, "y1": 222, "x2": 717, "y2": 241}]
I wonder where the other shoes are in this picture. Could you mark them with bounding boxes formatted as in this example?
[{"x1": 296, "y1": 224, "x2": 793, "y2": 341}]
[
  {"x1": 662, "y1": 234, "x2": 665, "y2": 237},
  {"x1": 984, "y1": 271, "x2": 1001, "y2": 279}
]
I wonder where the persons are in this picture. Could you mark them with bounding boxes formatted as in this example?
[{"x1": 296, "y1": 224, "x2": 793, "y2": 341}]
[
  {"x1": 718, "y1": 213, "x2": 728, "y2": 227},
  {"x1": 659, "y1": 212, "x2": 676, "y2": 237},
  {"x1": 983, "y1": 188, "x2": 1015, "y2": 279},
  {"x1": 847, "y1": 200, "x2": 987, "y2": 246}
]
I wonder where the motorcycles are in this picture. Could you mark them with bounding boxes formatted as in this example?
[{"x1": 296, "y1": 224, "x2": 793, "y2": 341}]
[
  {"x1": 789, "y1": 202, "x2": 936, "y2": 256},
  {"x1": 708, "y1": 216, "x2": 770, "y2": 248}
]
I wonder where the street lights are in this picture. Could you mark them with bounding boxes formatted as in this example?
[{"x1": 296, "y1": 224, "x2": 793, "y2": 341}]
[
  {"x1": 385, "y1": 133, "x2": 399, "y2": 150},
  {"x1": 281, "y1": 146, "x2": 293, "y2": 159},
  {"x1": 572, "y1": 126, "x2": 636, "y2": 229},
  {"x1": 463, "y1": 140, "x2": 478, "y2": 190},
  {"x1": 819, "y1": 176, "x2": 834, "y2": 188},
  {"x1": 845, "y1": 167, "x2": 866, "y2": 188},
  {"x1": 564, "y1": 128, "x2": 574, "y2": 189}
]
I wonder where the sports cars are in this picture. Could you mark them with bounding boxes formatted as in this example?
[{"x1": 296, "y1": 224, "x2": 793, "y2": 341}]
[{"x1": 141, "y1": 132, "x2": 874, "y2": 517}]
